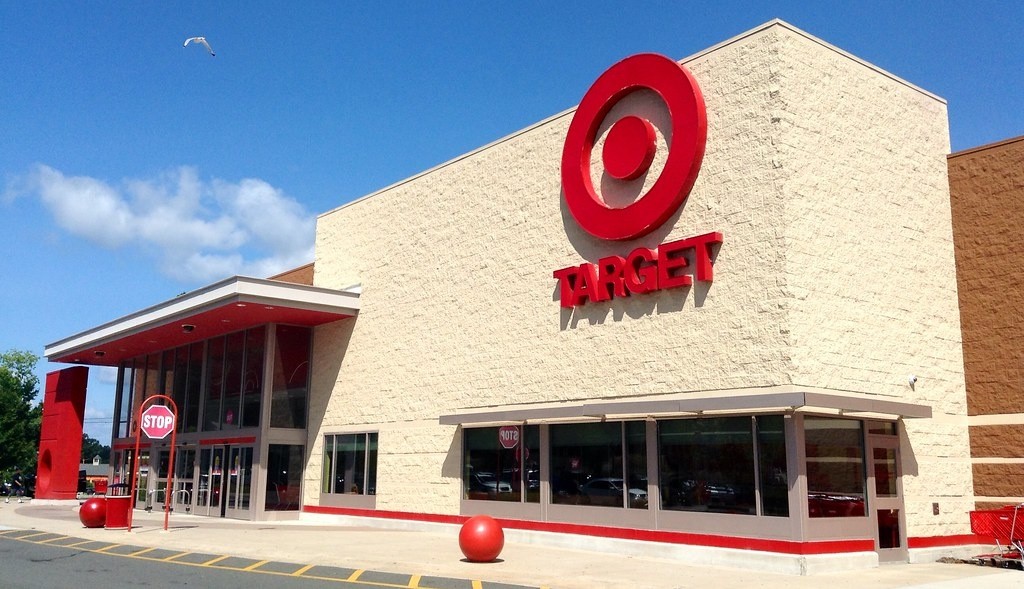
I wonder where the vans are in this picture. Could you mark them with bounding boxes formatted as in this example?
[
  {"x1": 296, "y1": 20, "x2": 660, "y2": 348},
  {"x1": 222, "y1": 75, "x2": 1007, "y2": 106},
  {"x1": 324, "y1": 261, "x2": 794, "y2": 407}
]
[{"x1": 507, "y1": 468, "x2": 540, "y2": 491}]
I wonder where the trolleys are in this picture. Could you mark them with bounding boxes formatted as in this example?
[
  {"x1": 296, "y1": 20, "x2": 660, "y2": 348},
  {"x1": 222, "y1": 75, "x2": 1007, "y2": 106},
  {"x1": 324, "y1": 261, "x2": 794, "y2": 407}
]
[{"x1": 968, "y1": 503, "x2": 1024, "y2": 571}]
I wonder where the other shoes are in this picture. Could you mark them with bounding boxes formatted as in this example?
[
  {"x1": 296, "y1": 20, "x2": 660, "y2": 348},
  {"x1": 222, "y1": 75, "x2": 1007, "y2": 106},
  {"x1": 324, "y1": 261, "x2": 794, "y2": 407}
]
[
  {"x1": 5, "y1": 498, "x2": 10, "y2": 504},
  {"x1": 17, "y1": 499, "x2": 23, "y2": 503}
]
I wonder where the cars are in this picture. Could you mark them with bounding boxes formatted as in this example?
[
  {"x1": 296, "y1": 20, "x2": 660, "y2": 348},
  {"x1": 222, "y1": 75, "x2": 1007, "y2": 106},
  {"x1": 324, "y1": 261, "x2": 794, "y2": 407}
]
[
  {"x1": 2, "y1": 480, "x2": 23, "y2": 496},
  {"x1": 188, "y1": 482, "x2": 219, "y2": 504},
  {"x1": 580, "y1": 478, "x2": 647, "y2": 509}
]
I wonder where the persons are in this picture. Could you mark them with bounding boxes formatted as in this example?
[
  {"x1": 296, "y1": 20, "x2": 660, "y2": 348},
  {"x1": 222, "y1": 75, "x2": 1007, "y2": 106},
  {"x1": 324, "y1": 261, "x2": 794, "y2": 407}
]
[{"x1": 5, "y1": 468, "x2": 23, "y2": 503}]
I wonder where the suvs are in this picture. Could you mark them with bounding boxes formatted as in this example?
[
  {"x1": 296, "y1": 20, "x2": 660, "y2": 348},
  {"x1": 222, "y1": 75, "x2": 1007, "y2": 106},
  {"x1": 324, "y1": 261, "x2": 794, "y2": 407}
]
[{"x1": 469, "y1": 471, "x2": 512, "y2": 499}]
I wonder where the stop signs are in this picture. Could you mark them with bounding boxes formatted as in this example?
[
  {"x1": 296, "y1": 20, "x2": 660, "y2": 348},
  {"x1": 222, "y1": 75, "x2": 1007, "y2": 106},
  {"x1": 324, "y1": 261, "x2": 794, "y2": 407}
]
[
  {"x1": 500, "y1": 425, "x2": 519, "y2": 449},
  {"x1": 141, "y1": 404, "x2": 175, "y2": 439}
]
